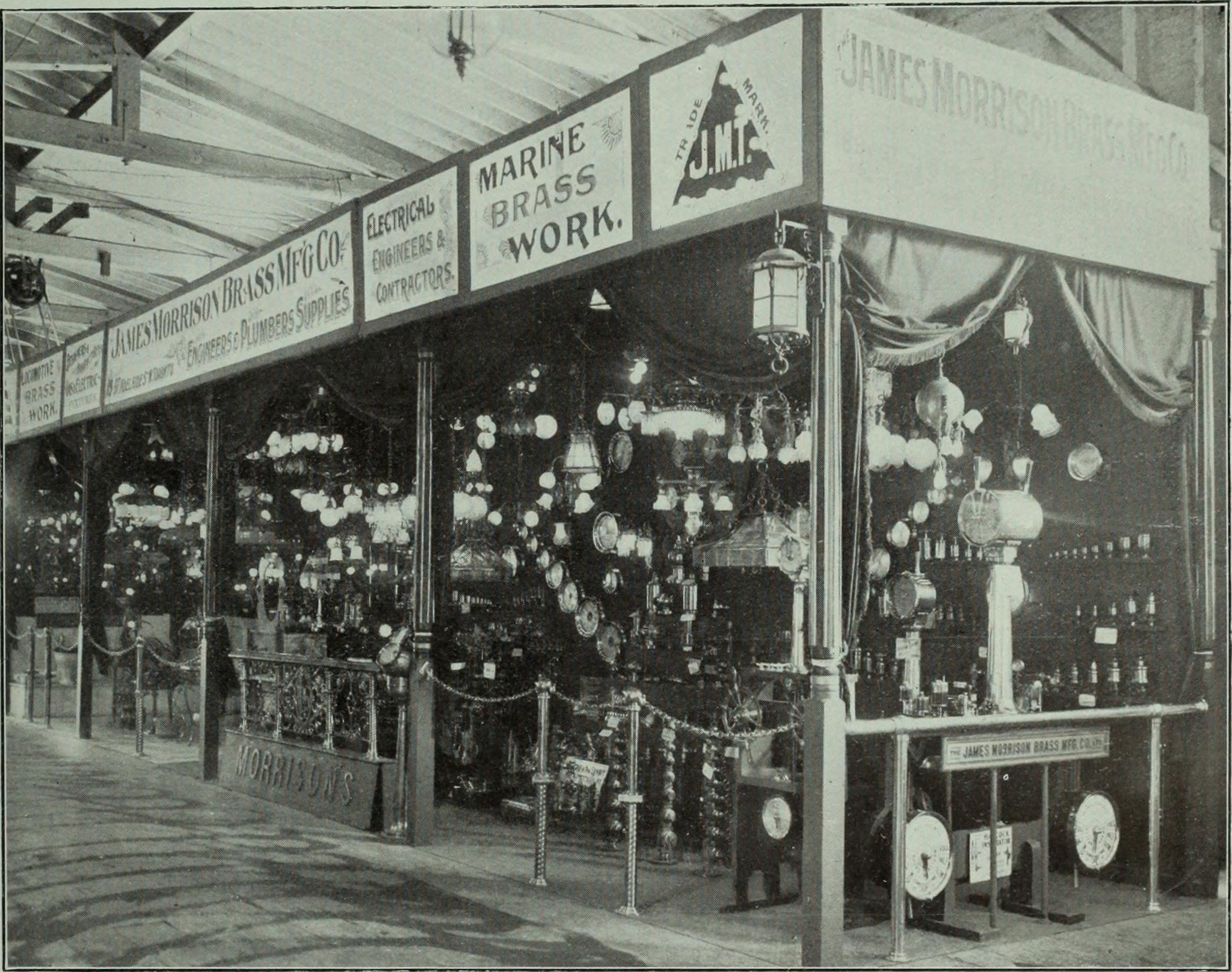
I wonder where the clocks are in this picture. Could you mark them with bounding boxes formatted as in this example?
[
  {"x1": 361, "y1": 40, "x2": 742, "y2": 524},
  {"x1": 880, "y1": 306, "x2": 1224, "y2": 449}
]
[
  {"x1": 761, "y1": 795, "x2": 797, "y2": 839},
  {"x1": 905, "y1": 807, "x2": 955, "y2": 901},
  {"x1": 1070, "y1": 788, "x2": 1122, "y2": 871}
]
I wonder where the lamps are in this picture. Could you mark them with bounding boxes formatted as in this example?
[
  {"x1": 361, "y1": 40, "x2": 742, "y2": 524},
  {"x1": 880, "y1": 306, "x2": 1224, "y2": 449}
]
[{"x1": 14, "y1": 208, "x2": 1157, "y2": 718}]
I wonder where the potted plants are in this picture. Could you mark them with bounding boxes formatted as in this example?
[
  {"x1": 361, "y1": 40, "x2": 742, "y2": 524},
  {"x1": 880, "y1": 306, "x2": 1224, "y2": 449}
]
[{"x1": 54, "y1": 646, "x2": 79, "y2": 686}]
